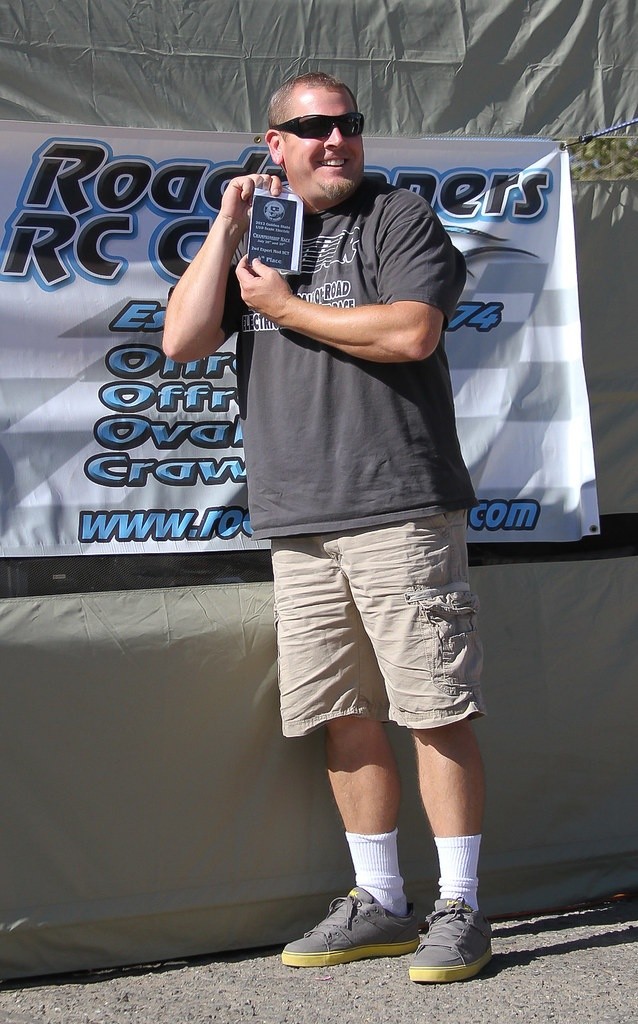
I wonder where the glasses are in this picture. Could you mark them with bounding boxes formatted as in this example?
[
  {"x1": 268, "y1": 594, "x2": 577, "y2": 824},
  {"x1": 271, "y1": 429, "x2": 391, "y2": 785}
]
[{"x1": 269, "y1": 112, "x2": 365, "y2": 139}]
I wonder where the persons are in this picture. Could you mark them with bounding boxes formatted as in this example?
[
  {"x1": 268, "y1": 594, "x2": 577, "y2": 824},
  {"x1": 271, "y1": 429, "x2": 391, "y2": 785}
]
[{"x1": 161, "y1": 70, "x2": 497, "y2": 984}]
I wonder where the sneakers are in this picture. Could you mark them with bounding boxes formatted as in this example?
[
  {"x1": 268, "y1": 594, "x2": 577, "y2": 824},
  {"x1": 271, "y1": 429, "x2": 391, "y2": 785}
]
[
  {"x1": 282, "y1": 887, "x2": 420, "y2": 967},
  {"x1": 408, "y1": 896, "x2": 493, "y2": 983}
]
